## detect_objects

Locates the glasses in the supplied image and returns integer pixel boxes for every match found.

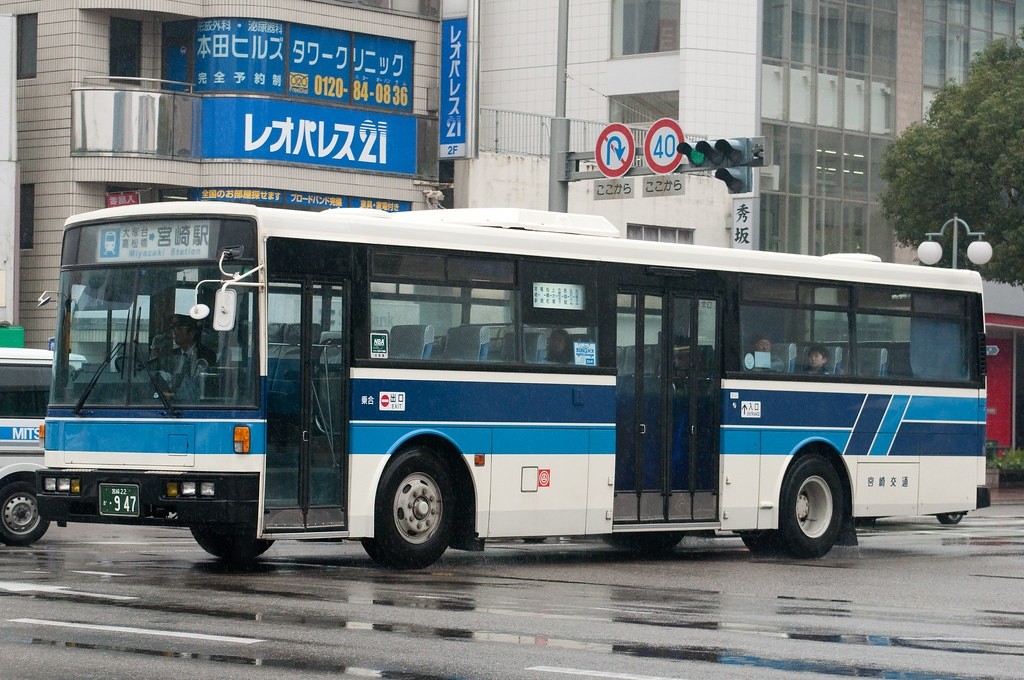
[150,345,164,350]
[171,326,191,333]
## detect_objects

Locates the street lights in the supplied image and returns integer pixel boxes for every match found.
[916,213,993,269]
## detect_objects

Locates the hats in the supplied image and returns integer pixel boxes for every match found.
[166,314,203,334]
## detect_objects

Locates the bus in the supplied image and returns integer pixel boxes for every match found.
[38,200,989,571]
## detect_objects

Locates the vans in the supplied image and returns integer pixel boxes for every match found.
[0,347,88,547]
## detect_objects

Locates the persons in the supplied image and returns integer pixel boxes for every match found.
[749,335,785,372]
[144,314,217,399]
[803,345,828,375]
[545,328,574,364]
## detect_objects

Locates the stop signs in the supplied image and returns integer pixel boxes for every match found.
[381,395,389,407]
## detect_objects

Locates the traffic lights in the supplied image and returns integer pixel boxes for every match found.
[675,137,754,195]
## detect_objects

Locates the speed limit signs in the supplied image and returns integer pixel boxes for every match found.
[643,118,684,175]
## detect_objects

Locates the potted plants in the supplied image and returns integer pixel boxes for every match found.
[986,460,1000,489]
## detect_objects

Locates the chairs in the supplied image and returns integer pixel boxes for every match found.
[801,346,842,374]
[389,324,551,363]
[268,322,341,415]
[847,348,887,377]
[616,343,715,400]
[770,343,797,373]
